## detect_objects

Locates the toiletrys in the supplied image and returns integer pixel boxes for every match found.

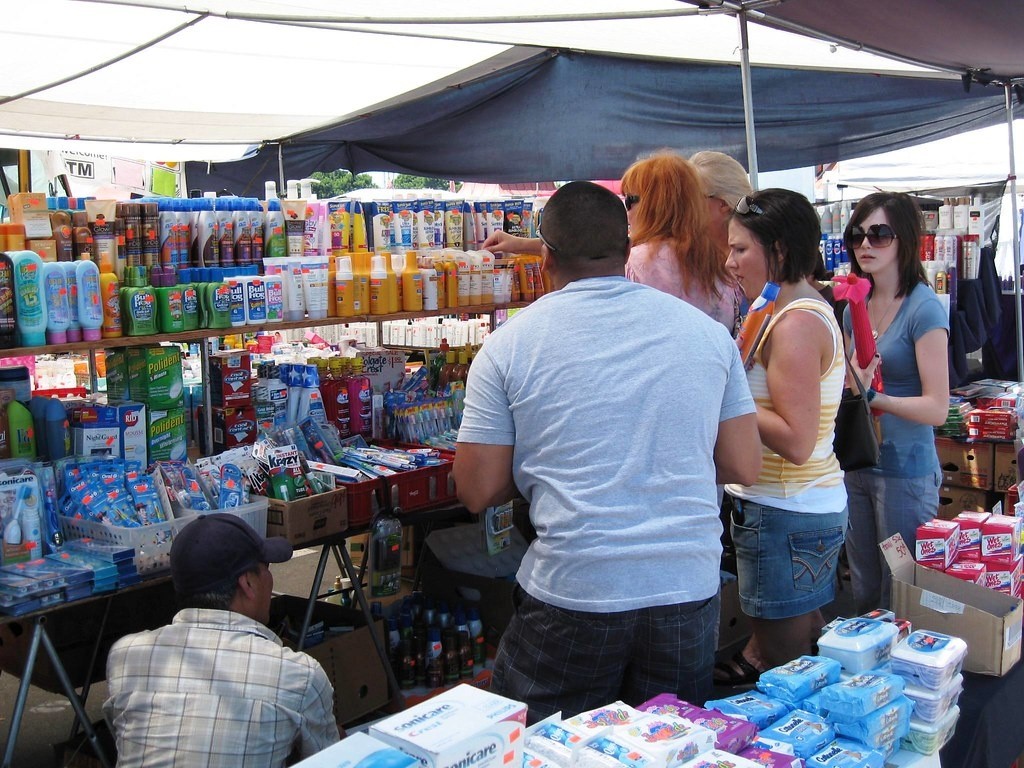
[916,197,986,310]
[816,196,853,287]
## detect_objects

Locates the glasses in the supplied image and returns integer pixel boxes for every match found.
[624,193,640,211]
[733,194,763,219]
[534,208,558,255]
[844,223,898,249]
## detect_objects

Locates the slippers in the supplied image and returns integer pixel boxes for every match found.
[712,651,767,683]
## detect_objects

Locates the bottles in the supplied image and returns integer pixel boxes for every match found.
[183,386,191,448]
[998,271,1013,290]
[433,338,472,390]
[191,385,202,446]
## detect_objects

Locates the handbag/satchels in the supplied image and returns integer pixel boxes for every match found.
[834,353,882,471]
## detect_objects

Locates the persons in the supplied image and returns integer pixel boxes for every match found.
[105,514,341,768]
[839,191,951,616]
[452,151,848,728]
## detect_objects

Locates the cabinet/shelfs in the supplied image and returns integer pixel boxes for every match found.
[0,233,966,768]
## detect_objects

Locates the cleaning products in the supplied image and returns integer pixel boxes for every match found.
[0,194,556,616]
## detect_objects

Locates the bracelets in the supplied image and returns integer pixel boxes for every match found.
[867,389,876,403]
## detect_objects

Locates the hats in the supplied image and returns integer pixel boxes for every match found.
[171,514,294,591]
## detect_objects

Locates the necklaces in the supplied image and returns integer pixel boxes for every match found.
[870,282,901,340]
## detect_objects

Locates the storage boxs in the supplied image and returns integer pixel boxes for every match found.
[262,594,526,768]
[881,534,1021,676]
[50,441,455,574]
[930,435,1015,522]
